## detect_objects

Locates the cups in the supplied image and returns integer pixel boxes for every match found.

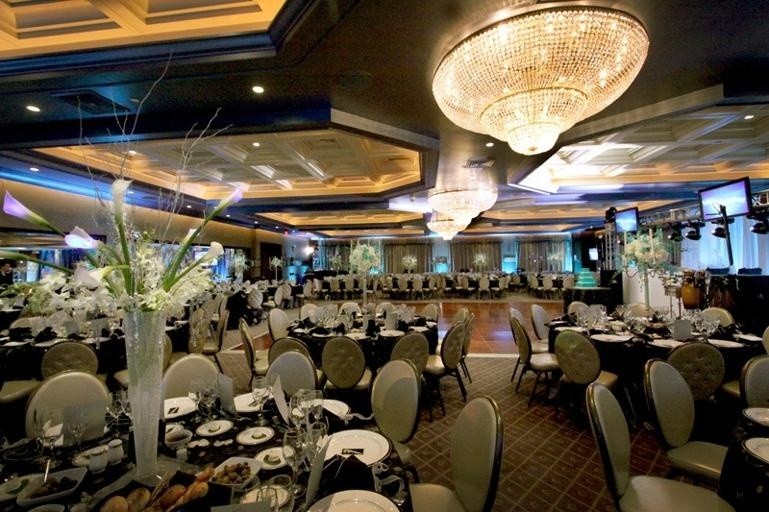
[310,391,324,422]
[268,474,295,512]
[232,475,264,505]
[303,423,329,467]
[123,390,133,421]
[106,391,127,423]
[256,488,280,512]
[62,408,89,451]
[87,448,108,475]
[108,438,124,466]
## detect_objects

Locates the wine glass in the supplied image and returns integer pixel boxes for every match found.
[36,408,66,468]
[200,379,218,422]
[288,395,310,447]
[284,428,308,500]
[188,380,205,423]
[298,388,315,427]
[550,305,763,349]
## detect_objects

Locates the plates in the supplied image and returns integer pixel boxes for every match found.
[236,426,274,446]
[163,397,197,420]
[240,484,292,510]
[16,468,87,506]
[307,490,401,512]
[319,429,393,468]
[290,303,431,340]
[1,474,41,502]
[253,379,271,425]
[206,457,262,489]
[741,437,769,464]
[291,399,351,418]
[39,423,109,447]
[71,444,110,467]
[91,478,211,512]
[1,331,125,347]
[232,385,273,412]
[256,446,296,471]
[742,407,769,427]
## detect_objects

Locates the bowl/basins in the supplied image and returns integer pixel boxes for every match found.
[165,424,185,433]
[196,420,234,436]
[166,430,194,448]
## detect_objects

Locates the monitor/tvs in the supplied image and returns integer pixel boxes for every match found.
[588,248,599,261]
[614,207,639,233]
[698,177,752,222]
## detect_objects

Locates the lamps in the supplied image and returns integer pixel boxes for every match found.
[427,186,499,241]
[431,0,651,156]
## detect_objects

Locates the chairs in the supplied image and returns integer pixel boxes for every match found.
[324,267,574,300]
[241,278,323,325]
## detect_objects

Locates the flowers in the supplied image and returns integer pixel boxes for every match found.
[1,46,253,395]
[619,226,673,273]
[348,243,381,272]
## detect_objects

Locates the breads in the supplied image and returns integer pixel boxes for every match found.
[100,465,213,512]
